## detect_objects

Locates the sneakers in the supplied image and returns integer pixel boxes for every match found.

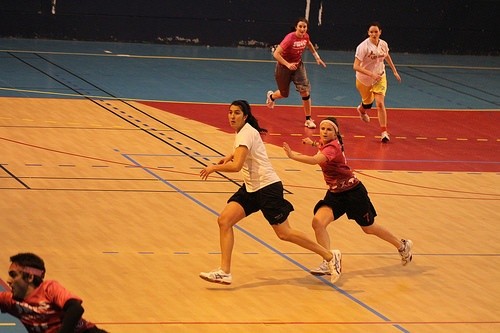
[324,250,344,283]
[357,105,370,123]
[266,90,275,110]
[381,131,390,143]
[310,260,330,276]
[398,239,413,267]
[197,267,233,285]
[305,117,316,129]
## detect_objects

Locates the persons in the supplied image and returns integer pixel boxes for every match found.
[199,99,342,285]
[266,19,326,129]
[283,117,412,276]
[304,0,323,50]
[0,253,110,333]
[353,21,400,141]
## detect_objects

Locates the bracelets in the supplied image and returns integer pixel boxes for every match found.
[312,140,316,148]
